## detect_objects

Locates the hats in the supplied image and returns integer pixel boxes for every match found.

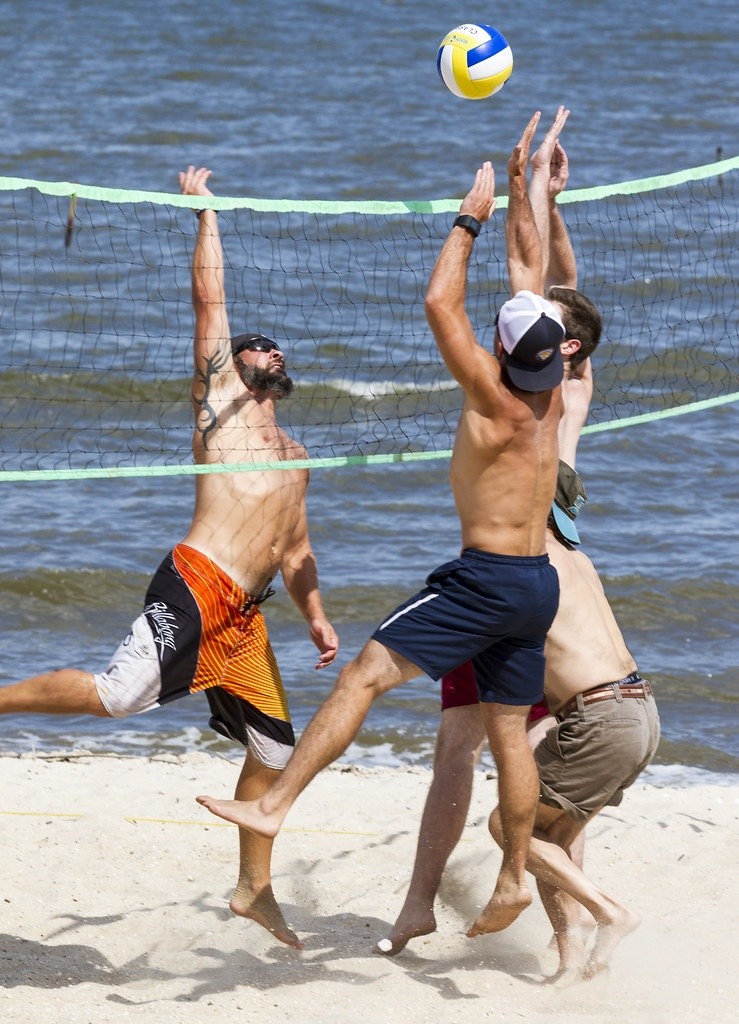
[550,458,586,545]
[498,290,566,392]
[231,334,266,351]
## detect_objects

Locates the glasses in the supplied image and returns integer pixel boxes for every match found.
[234,337,281,359]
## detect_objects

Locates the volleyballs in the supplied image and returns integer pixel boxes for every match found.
[437,24,513,100]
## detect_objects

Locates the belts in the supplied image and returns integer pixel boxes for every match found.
[558,681,653,723]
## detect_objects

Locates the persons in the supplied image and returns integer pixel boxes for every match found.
[197,111,566,936]
[2,164,340,951]
[372,102,604,956]
[490,458,660,988]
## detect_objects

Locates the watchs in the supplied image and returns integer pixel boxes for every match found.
[453,214,483,239]
